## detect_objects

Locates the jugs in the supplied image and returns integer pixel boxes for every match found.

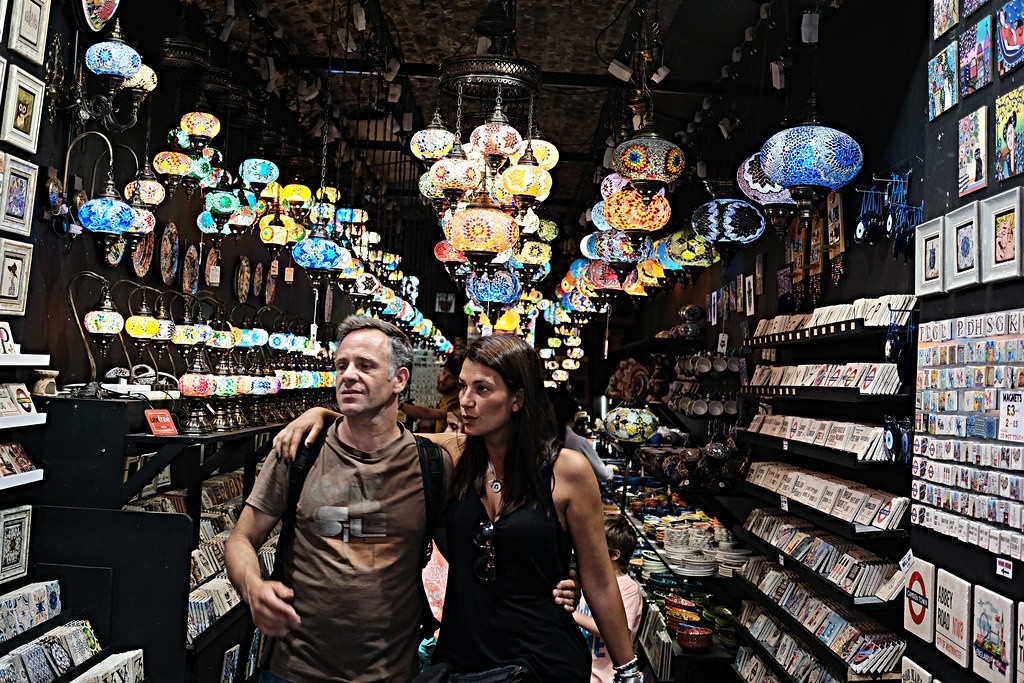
[641,503,687,516]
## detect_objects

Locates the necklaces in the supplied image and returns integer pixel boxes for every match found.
[488,460,503,492]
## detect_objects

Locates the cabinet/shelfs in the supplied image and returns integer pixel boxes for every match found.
[600,305,920,683]
[0,392,292,683]
[0,354,50,489]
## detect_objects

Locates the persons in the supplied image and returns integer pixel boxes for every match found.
[225,313,581,683]
[271,334,644,683]
[553,399,619,482]
[571,513,643,683]
[398,352,468,663]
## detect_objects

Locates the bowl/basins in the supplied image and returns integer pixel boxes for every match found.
[629,544,743,652]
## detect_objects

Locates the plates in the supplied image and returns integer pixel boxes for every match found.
[644,510,752,576]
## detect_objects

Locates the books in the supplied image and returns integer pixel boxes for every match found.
[733,294,1024,683]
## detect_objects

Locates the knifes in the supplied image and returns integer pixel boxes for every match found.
[713,296,715,321]
[747,280,752,311]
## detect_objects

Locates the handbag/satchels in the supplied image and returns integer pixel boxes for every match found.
[440,665,528,683]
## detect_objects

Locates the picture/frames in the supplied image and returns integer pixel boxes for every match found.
[0,153,39,237]
[1,64,46,155]
[0,237,34,316]
[915,215,944,298]
[944,200,979,293]
[8,0,50,66]
[0,504,33,583]
[981,186,1023,284]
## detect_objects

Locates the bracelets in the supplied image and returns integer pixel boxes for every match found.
[398,403,403,410]
[612,654,644,683]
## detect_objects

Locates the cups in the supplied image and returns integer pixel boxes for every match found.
[668,351,740,416]
[635,445,681,470]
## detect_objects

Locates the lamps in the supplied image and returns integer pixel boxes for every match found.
[46,0,866,398]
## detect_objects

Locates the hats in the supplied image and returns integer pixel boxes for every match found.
[440,359,462,376]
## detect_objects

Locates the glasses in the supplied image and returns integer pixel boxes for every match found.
[472,522,496,582]
